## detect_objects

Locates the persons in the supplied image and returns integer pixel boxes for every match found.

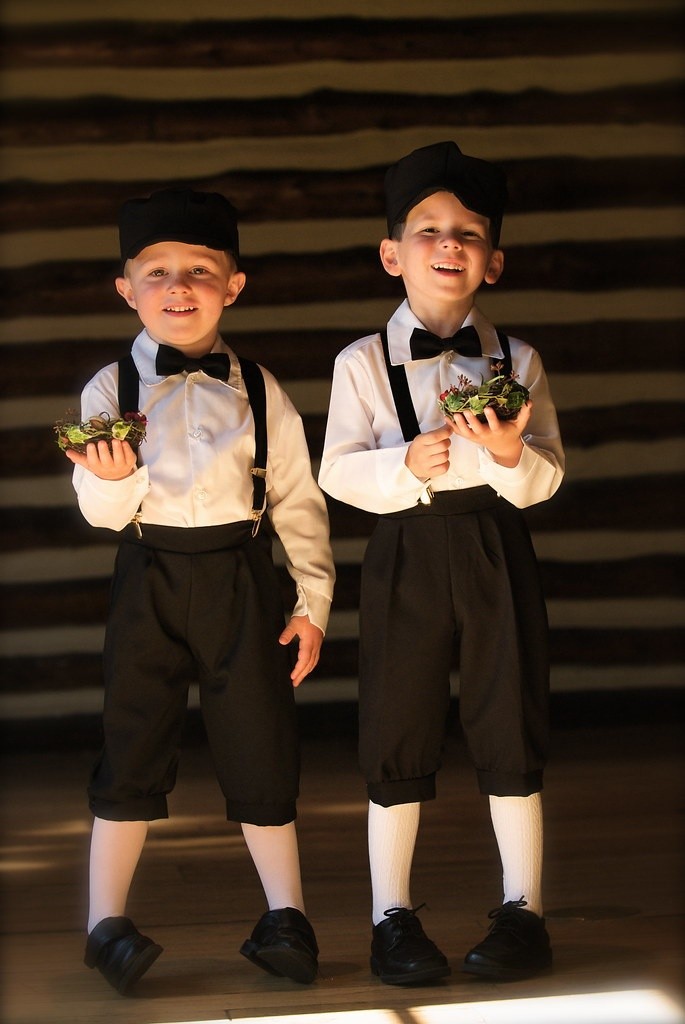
[65,192,338,993]
[316,140,565,987]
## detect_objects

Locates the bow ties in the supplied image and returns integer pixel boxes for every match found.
[155,344,231,384]
[410,324,483,362]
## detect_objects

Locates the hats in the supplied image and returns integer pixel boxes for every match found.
[381,140,512,247]
[115,185,239,274]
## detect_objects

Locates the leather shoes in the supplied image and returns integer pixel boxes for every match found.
[463,895,553,971]
[239,907,319,986]
[369,901,451,984]
[82,916,162,994]
